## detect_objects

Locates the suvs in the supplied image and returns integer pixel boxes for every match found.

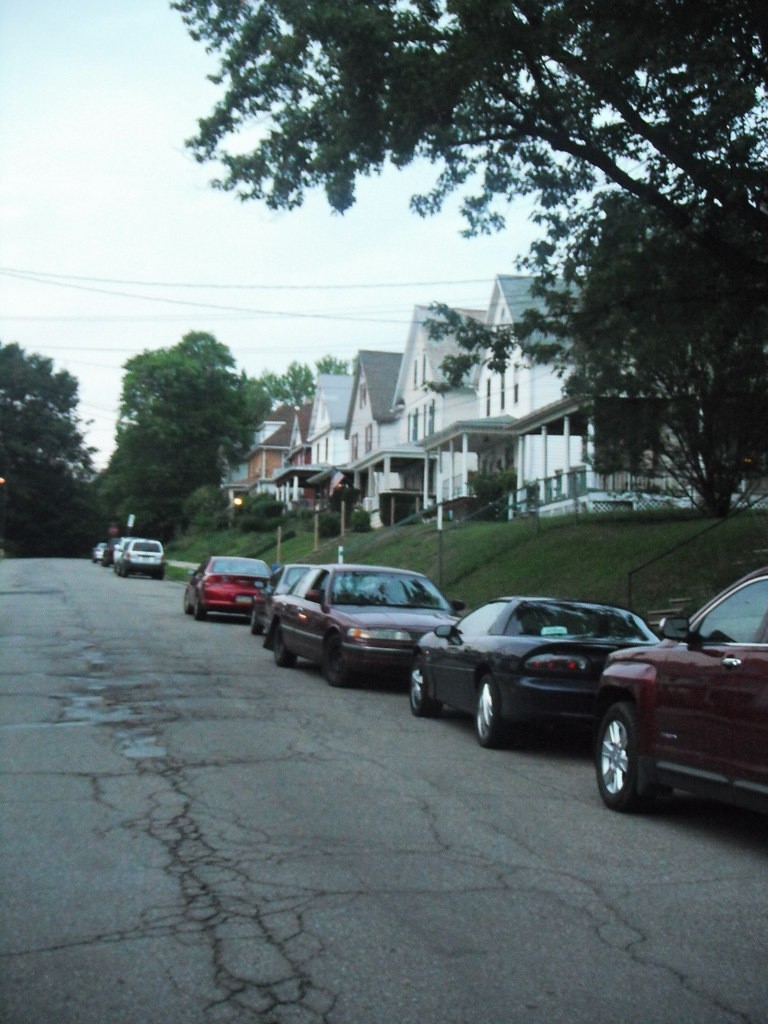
[116,539,166,579]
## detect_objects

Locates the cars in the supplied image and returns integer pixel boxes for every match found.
[182,556,273,620]
[249,566,314,632]
[266,564,465,689]
[409,593,659,748]
[590,564,768,817]
[92,537,135,567]
